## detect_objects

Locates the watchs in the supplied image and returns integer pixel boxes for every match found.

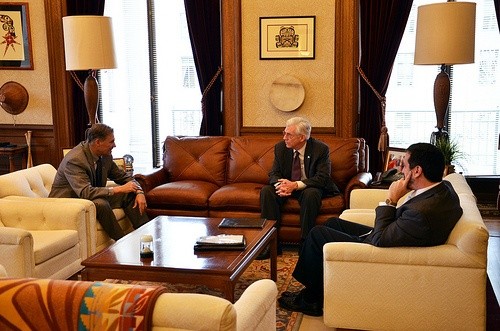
[385,198,397,206]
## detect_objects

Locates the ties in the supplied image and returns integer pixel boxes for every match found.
[95,160,102,186]
[292,151,301,181]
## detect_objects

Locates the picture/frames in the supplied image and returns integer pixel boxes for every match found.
[259,15,315,60]
[0,1,34,70]
[383,145,408,172]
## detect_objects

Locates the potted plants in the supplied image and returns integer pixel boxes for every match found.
[432,134,466,177]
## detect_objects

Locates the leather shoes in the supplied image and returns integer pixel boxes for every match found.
[278,291,324,317]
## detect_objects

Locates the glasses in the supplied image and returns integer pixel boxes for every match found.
[283,131,301,138]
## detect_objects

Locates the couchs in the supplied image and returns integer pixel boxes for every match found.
[1,134,489,330]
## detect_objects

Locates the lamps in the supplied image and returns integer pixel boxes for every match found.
[413,0,476,134]
[62,15,116,131]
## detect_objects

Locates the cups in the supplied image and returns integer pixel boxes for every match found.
[140,235,153,262]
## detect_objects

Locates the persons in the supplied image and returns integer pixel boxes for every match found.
[254,116,339,261]
[48,122,149,241]
[278,142,463,316]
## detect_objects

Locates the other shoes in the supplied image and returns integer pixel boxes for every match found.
[256,246,283,260]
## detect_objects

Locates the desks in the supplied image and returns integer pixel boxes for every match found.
[80,215,278,304]
[0,144,28,174]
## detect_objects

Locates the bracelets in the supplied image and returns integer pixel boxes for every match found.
[137,191,144,194]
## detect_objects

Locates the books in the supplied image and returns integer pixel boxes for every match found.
[219,218,266,228]
[194,234,246,250]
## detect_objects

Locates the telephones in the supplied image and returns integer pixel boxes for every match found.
[381,168,404,185]
